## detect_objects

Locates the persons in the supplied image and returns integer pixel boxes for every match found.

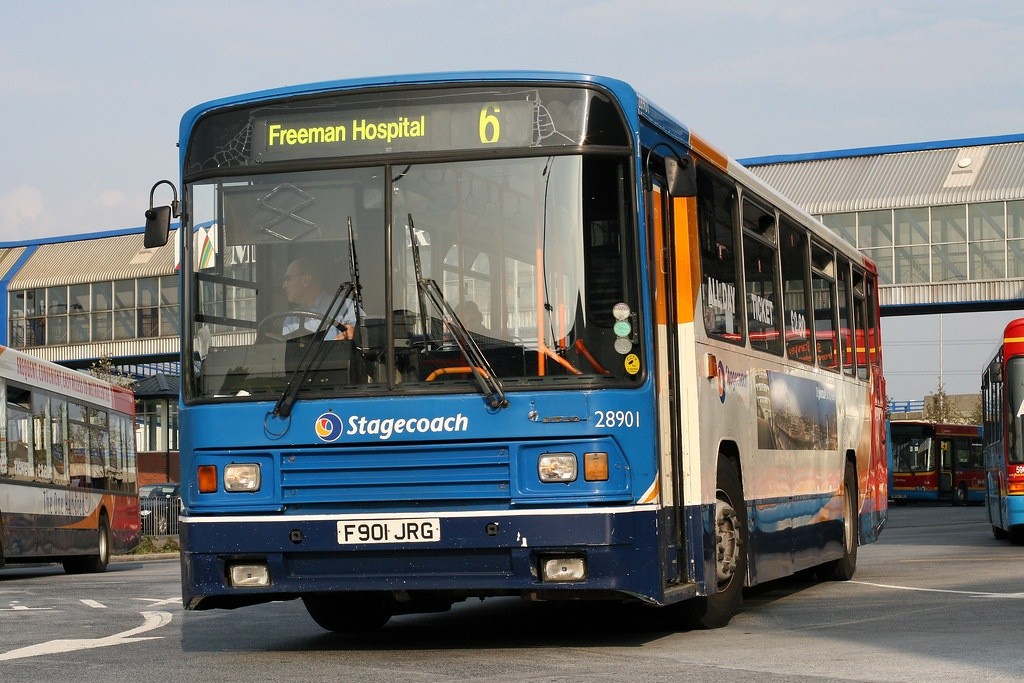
[283,256,367,340]
[454,301,491,336]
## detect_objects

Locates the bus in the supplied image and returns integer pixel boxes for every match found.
[723,329,877,383]
[981,318,1024,546]
[0,344,142,575]
[143,70,890,630]
[890,421,986,507]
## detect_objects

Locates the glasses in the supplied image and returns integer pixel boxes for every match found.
[284,272,304,280]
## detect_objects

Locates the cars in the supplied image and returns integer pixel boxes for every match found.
[138,483,180,535]
